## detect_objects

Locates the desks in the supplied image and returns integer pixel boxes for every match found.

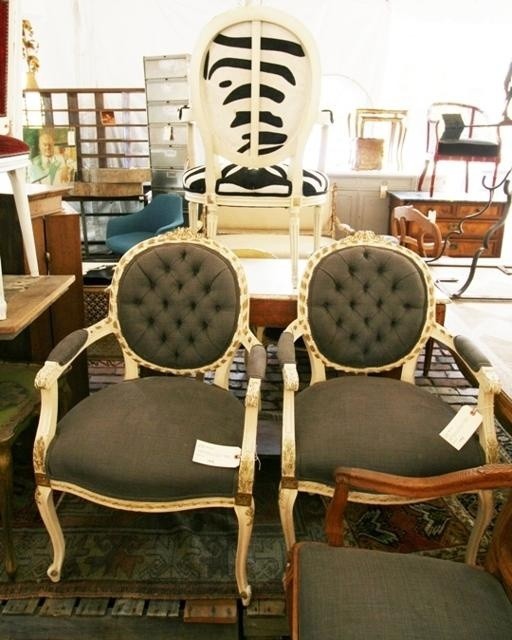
[344,108,409,174]
[0,359,73,578]
[239,256,447,383]
[0,275,76,339]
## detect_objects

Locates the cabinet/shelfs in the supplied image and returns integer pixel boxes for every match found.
[142,54,197,200]
[22,87,150,262]
[389,189,508,259]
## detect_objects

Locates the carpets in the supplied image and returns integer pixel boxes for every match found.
[0,404,511,599]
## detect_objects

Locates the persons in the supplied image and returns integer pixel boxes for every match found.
[31,133,67,186]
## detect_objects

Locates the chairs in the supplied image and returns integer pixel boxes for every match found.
[418,100,502,197]
[33,226,269,605]
[181,9,333,287]
[275,230,502,564]
[0,9,42,321]
[282,463,512,640]
